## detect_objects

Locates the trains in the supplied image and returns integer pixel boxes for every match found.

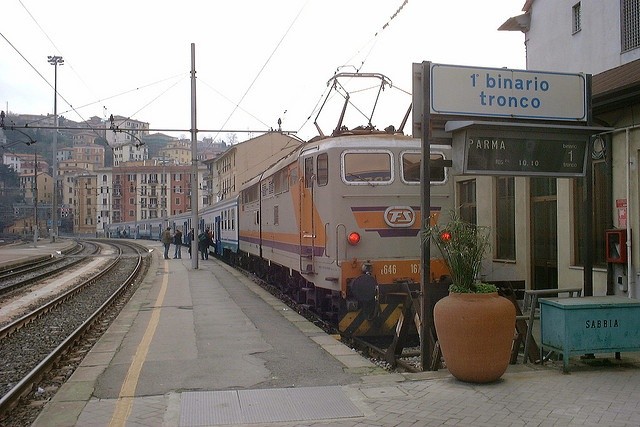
[104,129,456,338]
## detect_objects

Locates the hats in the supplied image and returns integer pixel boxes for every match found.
[167,227,171,229]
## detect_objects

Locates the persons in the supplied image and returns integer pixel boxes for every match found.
[161,227,173,259]
[188,230,192,259]
[198,228,212,260]
[206,227,213,249]
[173,229,182,259]
[123,230,126,238]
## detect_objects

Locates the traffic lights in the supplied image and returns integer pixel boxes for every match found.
[65,207,69,217]
[61,207,65,218]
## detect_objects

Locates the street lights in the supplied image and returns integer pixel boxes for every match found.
[47,53,64,243]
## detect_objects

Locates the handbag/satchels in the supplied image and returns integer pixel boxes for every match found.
[199,232,206,242]
